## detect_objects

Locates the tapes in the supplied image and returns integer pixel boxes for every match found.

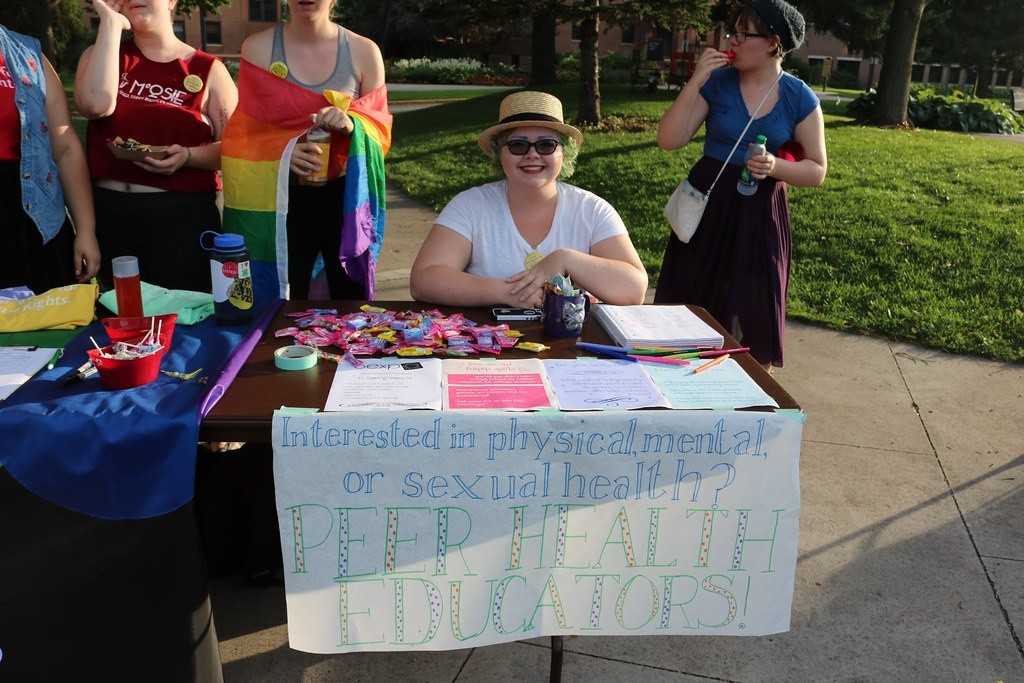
[274,345,318,370]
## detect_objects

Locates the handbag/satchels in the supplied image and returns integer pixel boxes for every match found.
[664,180,709,243]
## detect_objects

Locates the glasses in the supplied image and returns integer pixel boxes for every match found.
[502,138,561,155]
[724,30,764,43]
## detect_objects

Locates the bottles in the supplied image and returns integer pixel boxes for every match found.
[301,112,330,186]
[201,231,255,327]
[737,135,767,196]
[112,256,144,318]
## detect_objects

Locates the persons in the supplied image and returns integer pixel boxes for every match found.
[73,0,239,293]
[410,91,648,309]
[241,0,385,299]
[654,0,827,373]
[0,25,101,295]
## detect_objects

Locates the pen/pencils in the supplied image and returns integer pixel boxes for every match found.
[581,345,638,362]
[626,354,691,365]
[700,348,750,357]
[627,345,718,357]
[575,341,628,352]
[65,367,98,385]
[68,361,92,378]
[47,348,61,371]
[662,351,714,358]
[693,353,731,374]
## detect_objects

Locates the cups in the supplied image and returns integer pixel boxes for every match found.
[545,290,590,338]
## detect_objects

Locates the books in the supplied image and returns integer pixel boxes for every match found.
[591,304,724,349]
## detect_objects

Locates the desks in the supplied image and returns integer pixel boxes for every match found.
[0,299,803,683]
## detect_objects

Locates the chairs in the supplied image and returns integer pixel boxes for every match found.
[666,51,696,92]
[629,49,661,92]
[647,37,671,83]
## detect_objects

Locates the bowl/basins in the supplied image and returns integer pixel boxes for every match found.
[86,333,166,389]
[100,313,178,353]
[107,140,168,160]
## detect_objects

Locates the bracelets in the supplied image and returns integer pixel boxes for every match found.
[185,147,191,166]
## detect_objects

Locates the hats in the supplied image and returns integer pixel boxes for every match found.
[751,1,806,51]
[478,91,583,159]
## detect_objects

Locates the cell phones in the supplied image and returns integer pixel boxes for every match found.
[492,307,543,321]
